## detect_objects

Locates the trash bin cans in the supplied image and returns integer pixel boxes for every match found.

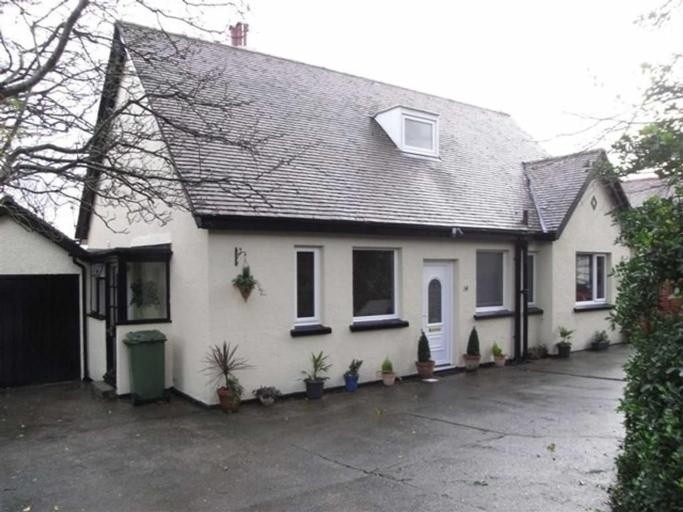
[122,329,171,406]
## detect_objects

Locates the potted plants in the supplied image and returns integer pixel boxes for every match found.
[252,384,286,409]
[551,324,578,359]
[229,244,266,305]
[340,357,364,394]
[459,325,484,373]
[376,352,404,388]
[588,330,613,352]
[413,327,438,381]
[194,338,261,415]
[487,340,510,368]
[300,349,330,401]
